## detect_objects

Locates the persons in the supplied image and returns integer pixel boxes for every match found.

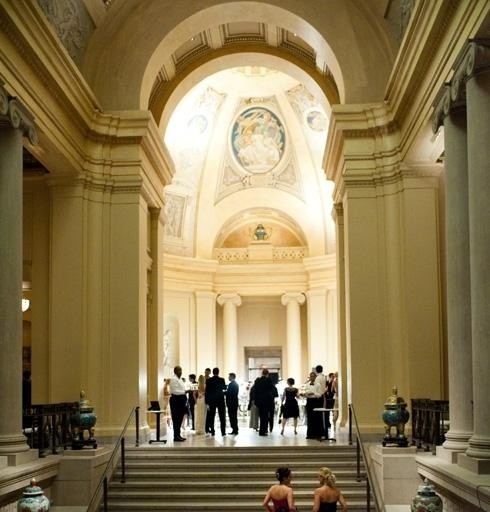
[312,467,347,512]
[163,366,339,441]
[262,467,298,512]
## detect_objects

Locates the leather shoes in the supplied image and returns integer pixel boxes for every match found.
[207,429,238,436]
[180,436,186,440]
[175,436,183,441]
